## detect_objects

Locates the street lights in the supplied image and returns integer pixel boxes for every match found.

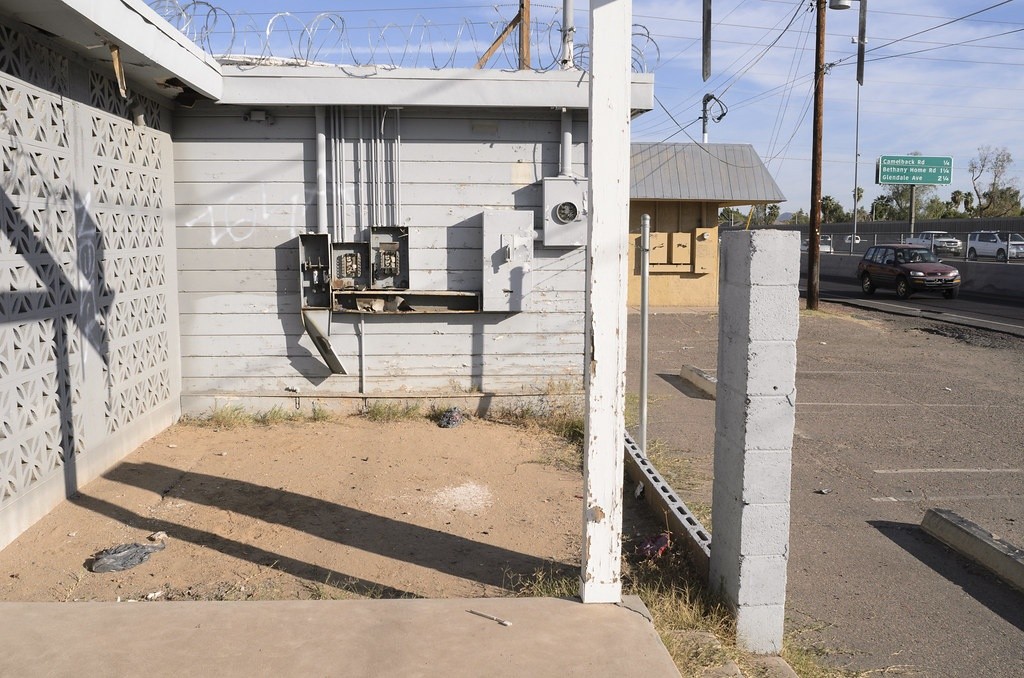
[806,0,852,311]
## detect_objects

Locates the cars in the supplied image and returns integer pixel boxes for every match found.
[800,234,834,255]
[845,235,861,244]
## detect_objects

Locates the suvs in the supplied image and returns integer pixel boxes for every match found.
[856,244,961,301]
[968,230,1024,262]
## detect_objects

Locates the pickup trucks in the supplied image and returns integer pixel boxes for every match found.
[906,230,963,256]
